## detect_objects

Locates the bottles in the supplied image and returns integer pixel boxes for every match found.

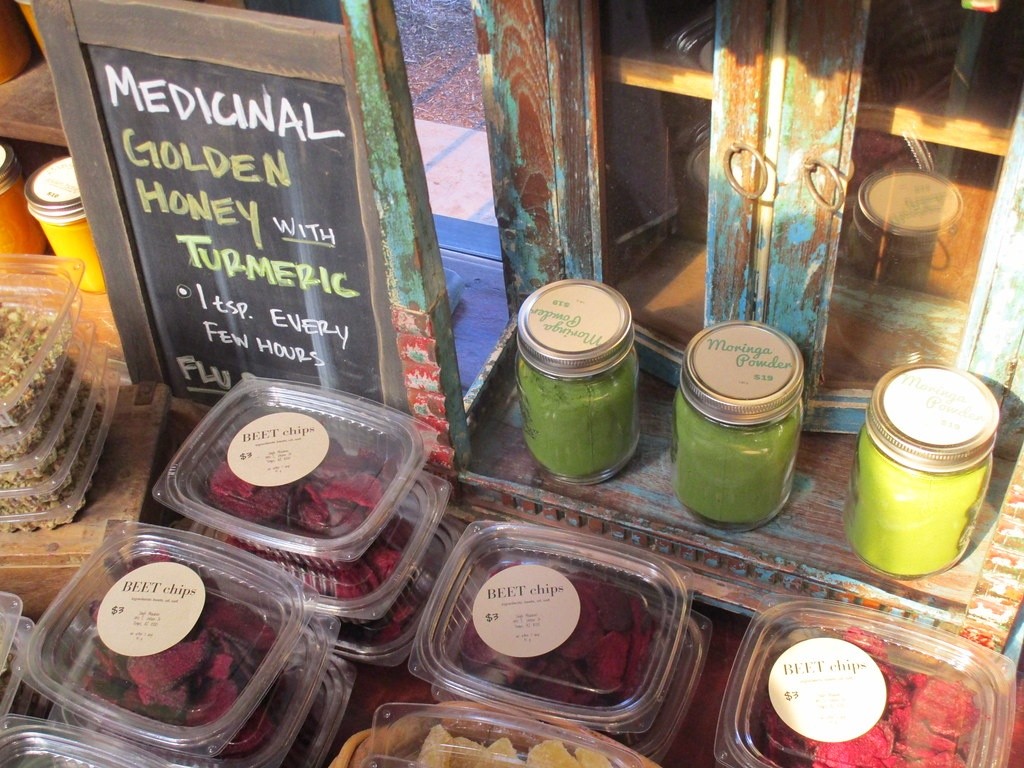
[671,320,807,532]
[1,0,110,296]
[845,360,1001,580]
[515,278,643,485]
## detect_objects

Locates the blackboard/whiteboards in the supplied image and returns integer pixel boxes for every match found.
[34,0,406,410]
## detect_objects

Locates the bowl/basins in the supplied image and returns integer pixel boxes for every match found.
[0,246,1019,768]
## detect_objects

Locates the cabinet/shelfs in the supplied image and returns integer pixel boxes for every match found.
[475,0,1024,434]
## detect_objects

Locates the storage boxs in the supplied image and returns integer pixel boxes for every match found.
[0,251,1017,768]
